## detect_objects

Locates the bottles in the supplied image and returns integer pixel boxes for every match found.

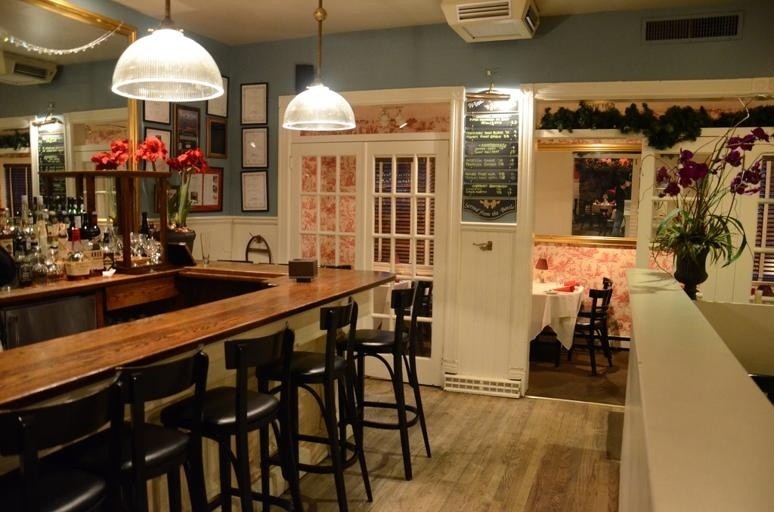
[14,194,96,249]
[68,227,114,277]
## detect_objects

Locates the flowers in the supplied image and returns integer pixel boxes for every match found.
[90,138,134,170]
[648,125,769,278]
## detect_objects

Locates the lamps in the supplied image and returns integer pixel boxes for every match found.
[33,103,59,128]
[107,0,224,104]
[281,1,357,132]
[465,66,511,102]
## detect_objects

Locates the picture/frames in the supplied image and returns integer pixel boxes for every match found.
[138,72,271,215]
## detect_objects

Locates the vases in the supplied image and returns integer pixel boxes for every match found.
[672,237,710,299]
[155,230,195,266]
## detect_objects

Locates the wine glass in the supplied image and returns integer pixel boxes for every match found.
[116,231,163,265]
[32,246,63,284]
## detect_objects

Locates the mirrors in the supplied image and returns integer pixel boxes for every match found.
[0,0,142,268]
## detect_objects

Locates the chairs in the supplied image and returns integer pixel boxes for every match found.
[556,276,612,377]
